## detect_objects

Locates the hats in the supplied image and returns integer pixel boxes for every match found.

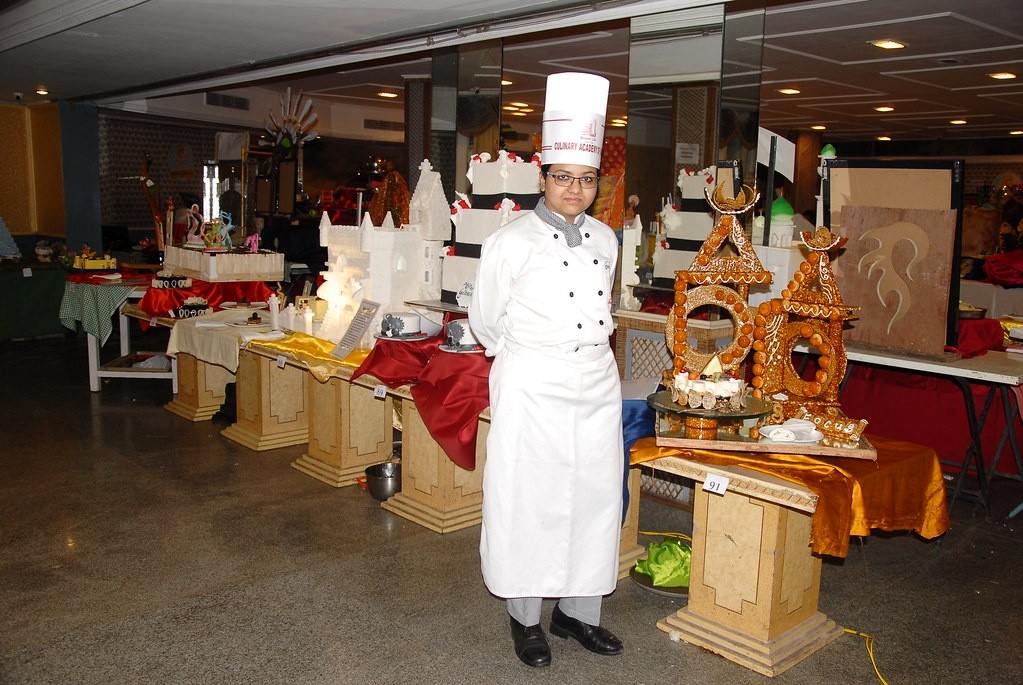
[541,72,610,169]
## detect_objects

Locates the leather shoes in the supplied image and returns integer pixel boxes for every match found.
[549,602,623,656]
[509,615,552,668]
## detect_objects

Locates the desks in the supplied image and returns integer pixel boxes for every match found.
[785,334,1023,522]
[65,270,263,394]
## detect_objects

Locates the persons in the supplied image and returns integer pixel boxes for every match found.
[467,142,624,668]
[289,199,328,287]
[1001,190,1023,235]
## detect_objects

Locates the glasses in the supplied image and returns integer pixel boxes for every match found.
[547,172,599,189]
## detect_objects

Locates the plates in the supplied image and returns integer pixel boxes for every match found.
[958,307,988,320]
[224,317,273,328]
[1003,313,1023,324]
[759,424,825,444]
[218,300,268,311]
